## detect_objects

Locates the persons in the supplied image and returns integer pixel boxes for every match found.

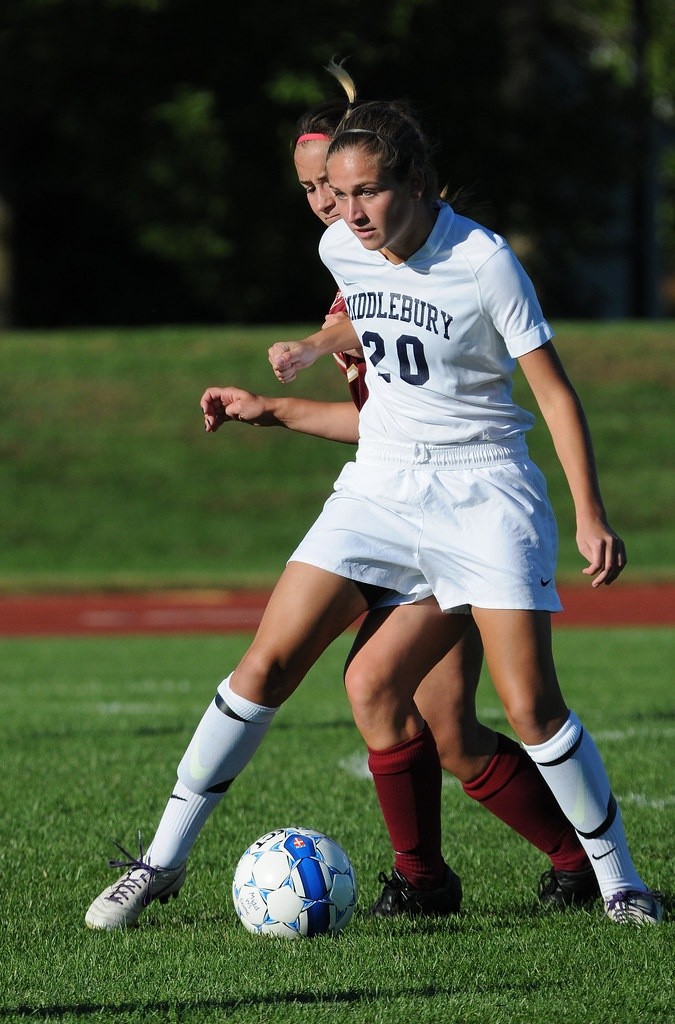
[75,91,673,933]
[200,106,607,927]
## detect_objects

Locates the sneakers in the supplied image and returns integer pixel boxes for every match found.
[531,865,600,912]
[603,885,668,929]
[84,829,187,930]
[366,869,463,917]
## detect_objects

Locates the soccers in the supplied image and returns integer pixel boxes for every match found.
[232,826,357,939]
